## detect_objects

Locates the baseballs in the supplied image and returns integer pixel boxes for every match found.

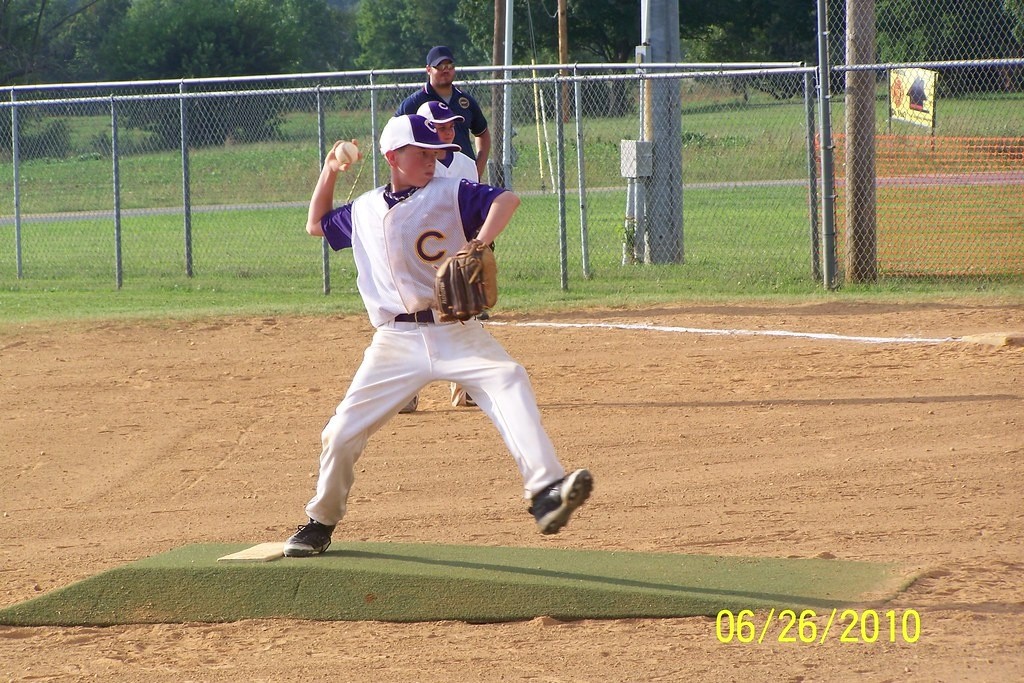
[334,142,359,164]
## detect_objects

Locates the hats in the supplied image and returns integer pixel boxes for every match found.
[379,114,462,156]
[416,101,465,124]
[426,46,454,67]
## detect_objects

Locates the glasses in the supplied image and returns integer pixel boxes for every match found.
[433,63,455,71]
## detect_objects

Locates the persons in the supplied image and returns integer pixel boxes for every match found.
[398,101,479,411]
[283,115,594,560]
[396,46,489,184]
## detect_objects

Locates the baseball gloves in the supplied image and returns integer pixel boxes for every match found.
[435,237,498,318]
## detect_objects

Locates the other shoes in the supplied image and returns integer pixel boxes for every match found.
[476,312,489,320]
[398,396,418,413]
[466,392,477,406]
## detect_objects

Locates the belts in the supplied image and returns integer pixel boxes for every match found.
[395,310,434,324]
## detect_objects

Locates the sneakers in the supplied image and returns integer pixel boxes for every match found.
[283,517,336,557]
[528,469,593,535]
